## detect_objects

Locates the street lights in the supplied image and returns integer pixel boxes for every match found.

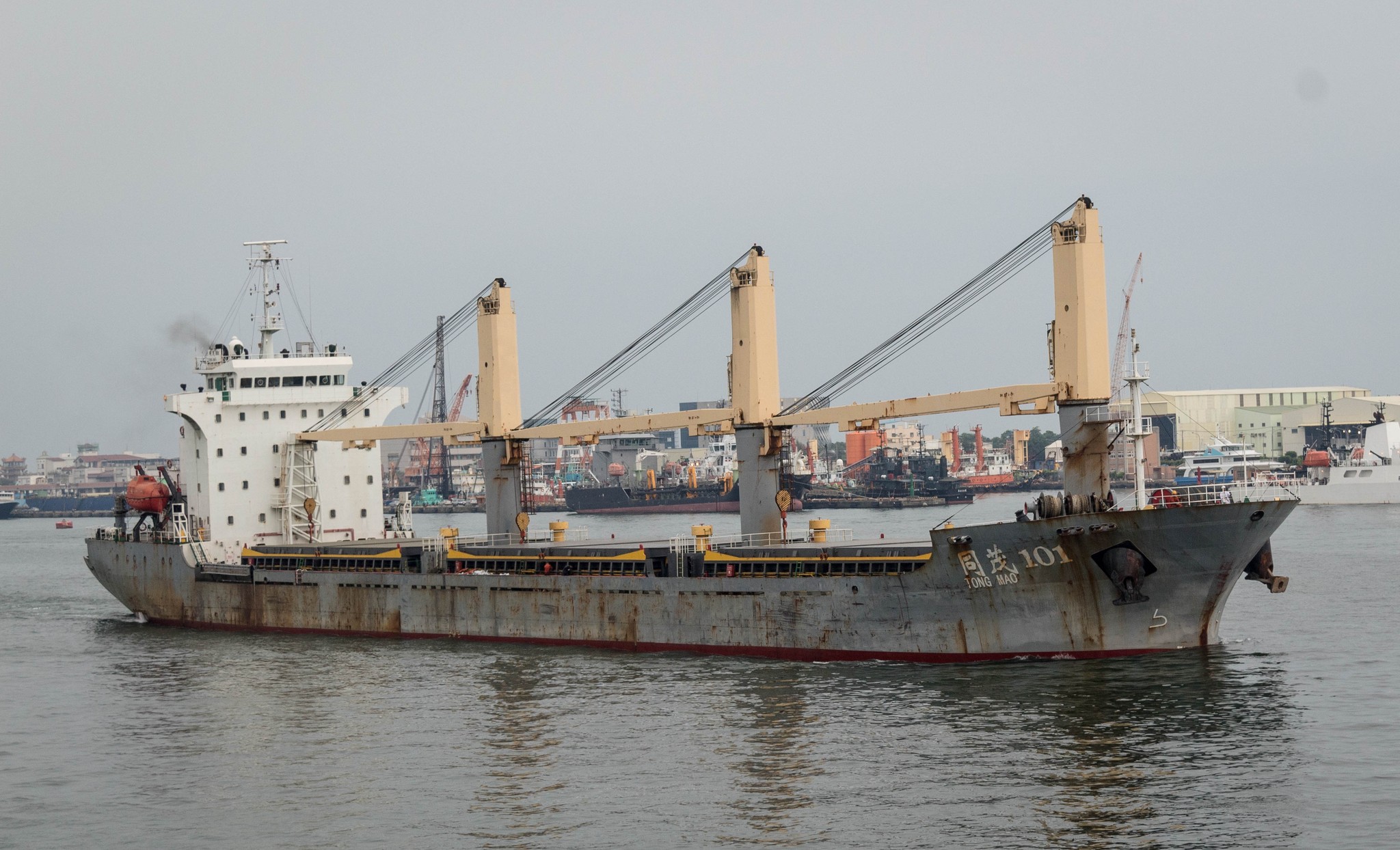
[1191,432,1201,452]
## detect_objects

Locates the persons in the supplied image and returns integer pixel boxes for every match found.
[1170,491,1182,507]
[544,562,552,575]
[1220,485,1232,504]
[561,564,573,576]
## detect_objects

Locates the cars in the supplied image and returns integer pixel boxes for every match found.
[388,500,399,506]
[451,499,465,506]
[437,500,451,505]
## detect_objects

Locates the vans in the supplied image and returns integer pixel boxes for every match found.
[465,497,478,504]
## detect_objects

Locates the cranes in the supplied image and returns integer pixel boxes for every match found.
[407,372,474,482]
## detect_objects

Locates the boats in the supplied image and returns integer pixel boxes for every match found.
[1229,398,1400,504]
[964,477,1033,494]
[78,237,1309,661]
[1174,426,1298,485]
[26,481,131,512]
[852,422,975,504]
[0,491,18,518]
[563,462,814,514]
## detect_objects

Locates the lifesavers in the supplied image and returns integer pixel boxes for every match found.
[1299,466,1303,470]
[1373,461,1376,466]
[180,427,184,436]
[1296,466,1299,470]
[167,459,172,468]
[140,523,147,531]
